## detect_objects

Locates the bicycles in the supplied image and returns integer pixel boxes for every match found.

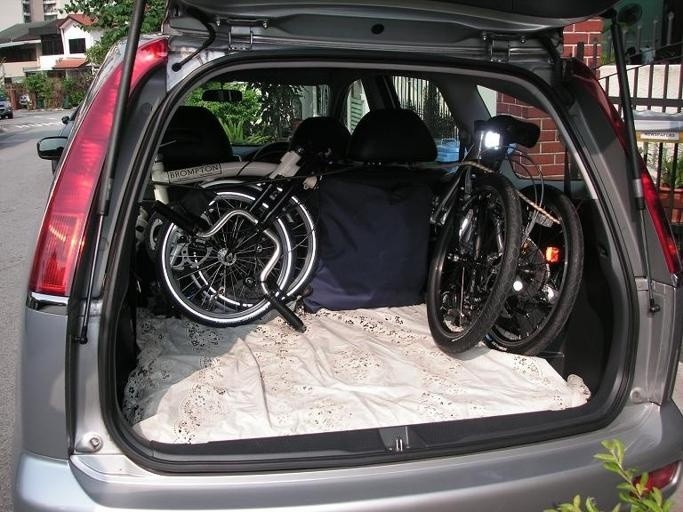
[424,116,584,356]
[144,137,337,333]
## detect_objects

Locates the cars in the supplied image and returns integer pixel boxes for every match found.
[14,0,683,512]
[0,95,13,119]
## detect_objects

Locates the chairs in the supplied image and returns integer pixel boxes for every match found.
[160,105,450,308]
